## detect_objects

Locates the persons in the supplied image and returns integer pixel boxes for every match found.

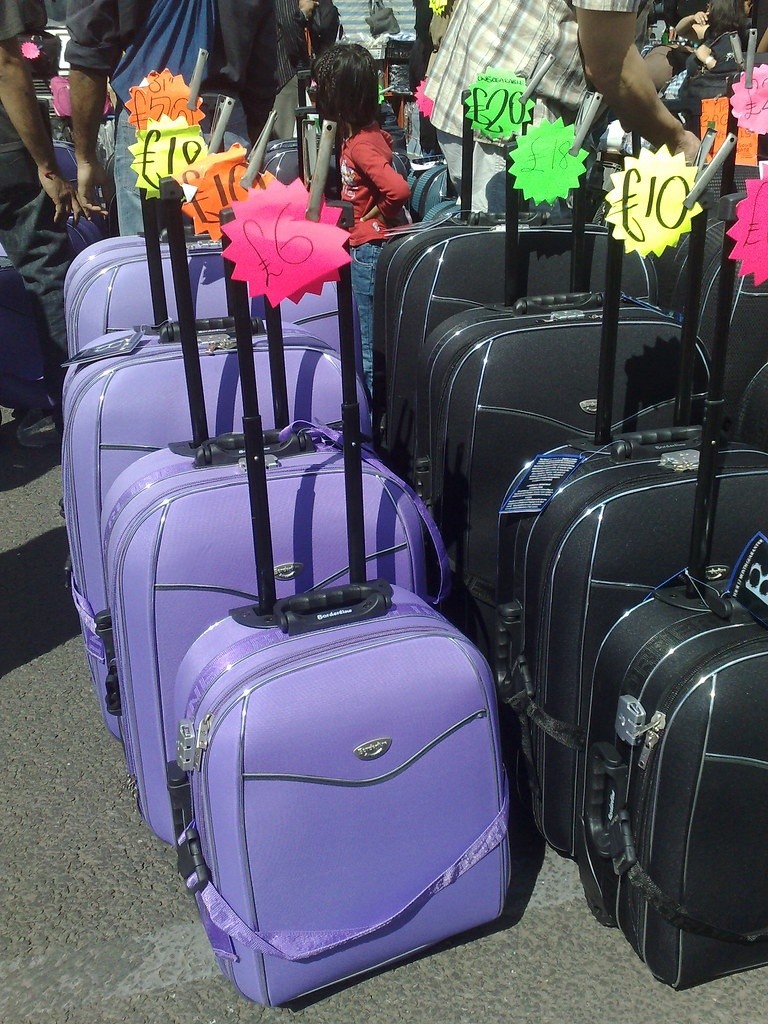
[0,0,768,438]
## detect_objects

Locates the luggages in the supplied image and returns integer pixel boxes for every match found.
[46,66,767,1009]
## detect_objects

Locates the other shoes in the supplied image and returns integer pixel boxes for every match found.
[17,415,59,450]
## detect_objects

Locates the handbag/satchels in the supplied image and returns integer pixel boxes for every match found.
[50,75,113,122]
[365,1,400,39]
